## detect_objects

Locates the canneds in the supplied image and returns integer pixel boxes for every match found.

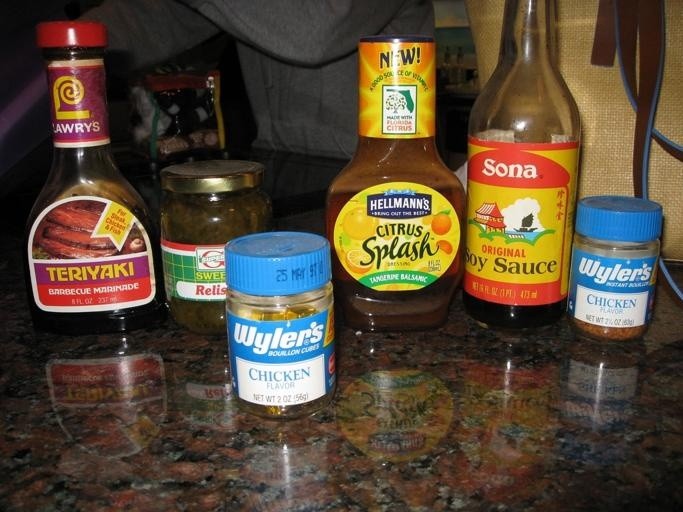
[158,159,279,336]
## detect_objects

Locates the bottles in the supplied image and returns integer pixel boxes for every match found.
[463,2,584,324]
[21,20,165,336]
[17,328,654,510]
[320,35,463,333]
